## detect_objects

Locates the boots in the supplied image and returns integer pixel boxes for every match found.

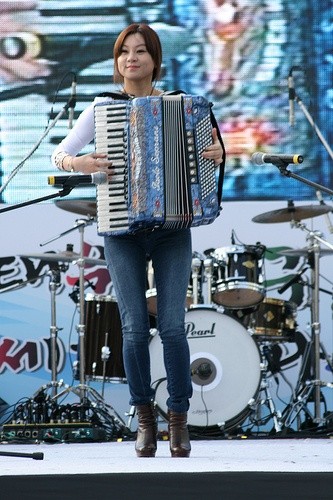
[168,409,191,457]
[135,401,157,457]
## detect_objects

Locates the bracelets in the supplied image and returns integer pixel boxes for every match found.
[68,157,78,173]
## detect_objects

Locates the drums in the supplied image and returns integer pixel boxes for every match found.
[208,243,267,309]
[74,292,130,384]
[144,306,265,437]
[222,297,298,342]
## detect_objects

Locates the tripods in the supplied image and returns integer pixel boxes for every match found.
[15,213,129,441]
[241,215,333,434]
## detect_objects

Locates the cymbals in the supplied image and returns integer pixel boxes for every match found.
[250,204,333,224]
[53,196,98,217]
[15,253,108,266]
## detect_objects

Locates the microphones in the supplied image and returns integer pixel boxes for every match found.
[251,152,303,165]
[316,190,333,233]
[288,76,295,127]
[68,75,77,129]
[48,172,109,187]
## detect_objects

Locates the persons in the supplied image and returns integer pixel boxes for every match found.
[51,24,223,457]
